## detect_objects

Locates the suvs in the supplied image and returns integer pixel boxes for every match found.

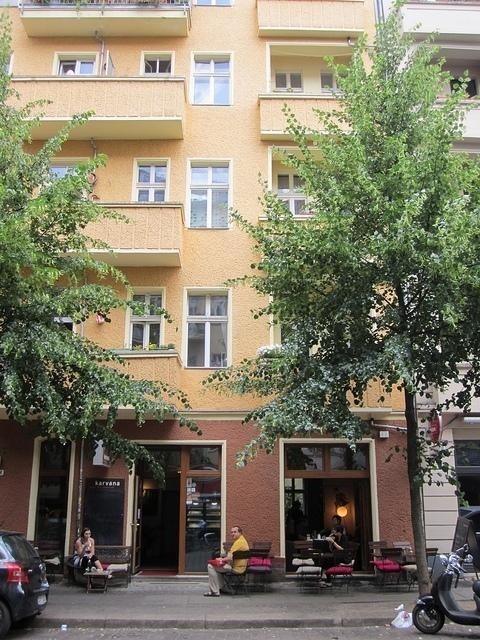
[0,530,50,640]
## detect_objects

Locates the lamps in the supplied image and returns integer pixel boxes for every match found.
[335,487,350,517]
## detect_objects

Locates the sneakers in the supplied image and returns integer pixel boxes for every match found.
[105,571,112,579]
[320,581,332,587]
[204,589,222,596]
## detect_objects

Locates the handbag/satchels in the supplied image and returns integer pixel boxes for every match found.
[72,554,88,567]
[208,560,226,567]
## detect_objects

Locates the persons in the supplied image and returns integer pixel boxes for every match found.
[204,526,249,597]
[329,515,344,537]
[321,525,352,588]
[73,527,112,579]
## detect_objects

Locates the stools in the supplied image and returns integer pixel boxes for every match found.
[83,571,112,595]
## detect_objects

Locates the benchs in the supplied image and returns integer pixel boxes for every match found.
[69,546,133,588]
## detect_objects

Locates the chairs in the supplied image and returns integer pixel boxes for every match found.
[369,541,438,592]
[291,535,359,595]
[213,542,274,598]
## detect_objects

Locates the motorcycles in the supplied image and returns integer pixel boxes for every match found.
[412,544,480,633]
